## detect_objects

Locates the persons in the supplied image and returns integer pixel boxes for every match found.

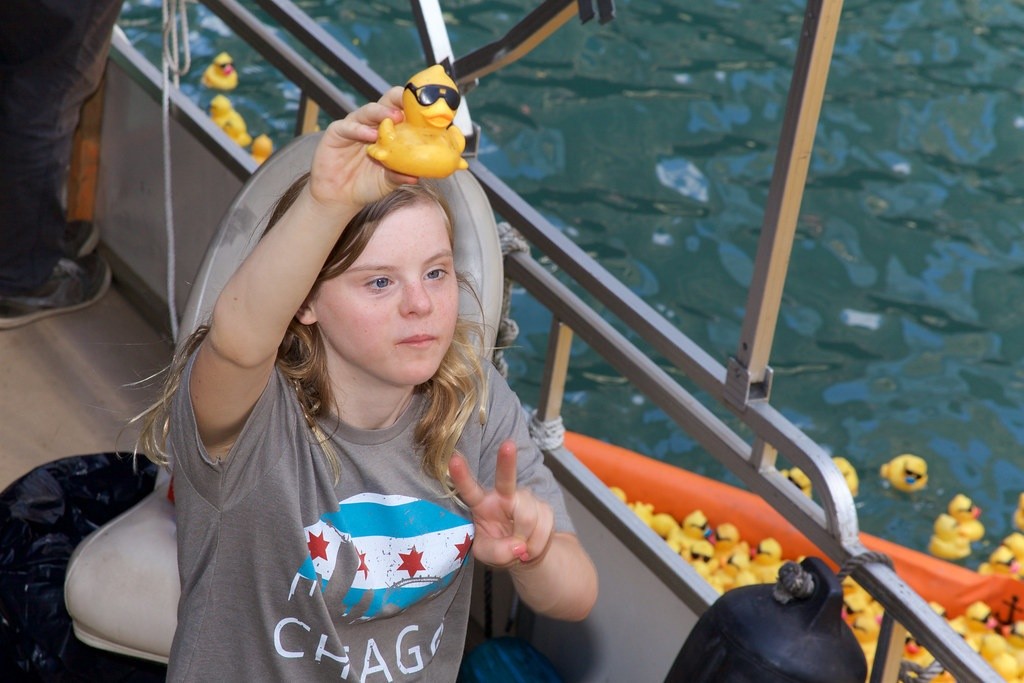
[113,87,597,683]
[1,1,112,334]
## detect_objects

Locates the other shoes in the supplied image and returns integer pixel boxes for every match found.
[0,217,114,330]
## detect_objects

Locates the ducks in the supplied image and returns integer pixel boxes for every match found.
[605,453,1024,683]
[198,51,273,167]
[368,65,469,180]
